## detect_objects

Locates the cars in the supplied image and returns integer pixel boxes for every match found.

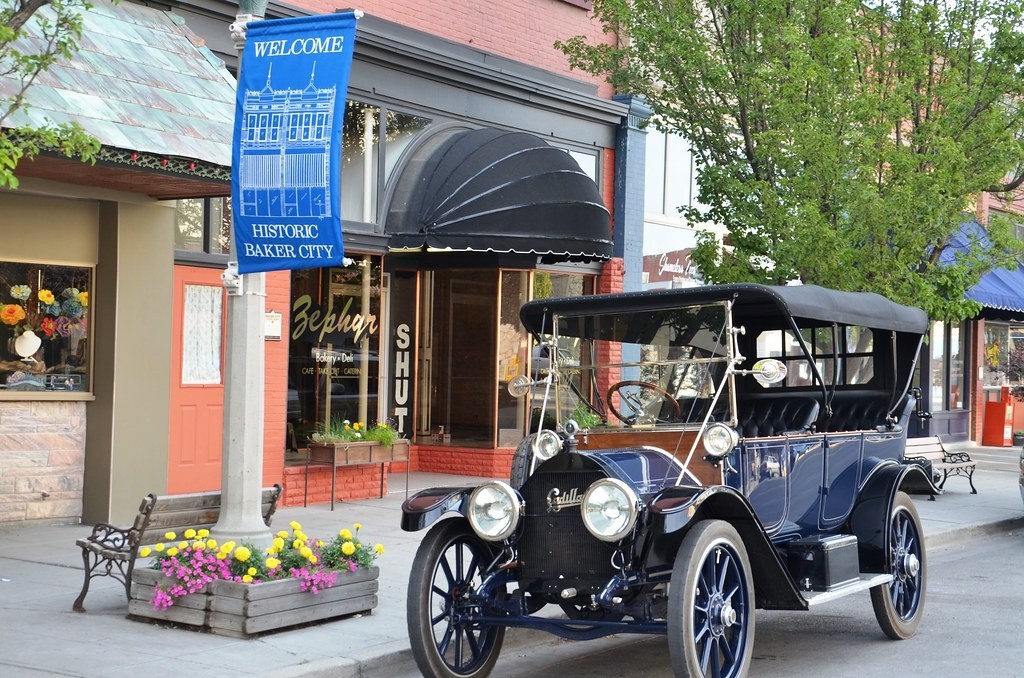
[398,282,948,676]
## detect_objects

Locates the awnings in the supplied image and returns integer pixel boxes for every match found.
[384,128,614,265]
[838,208,1024,322]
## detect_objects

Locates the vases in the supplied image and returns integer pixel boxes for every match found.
[125,565,379,639]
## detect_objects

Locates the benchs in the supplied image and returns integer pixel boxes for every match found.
[73,484,283,613]
[702,390,898,439]
[904,434,976,501]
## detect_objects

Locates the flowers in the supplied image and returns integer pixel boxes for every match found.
[140,520,384,613]
[307,410,399,449]
[0,284,88,338]
